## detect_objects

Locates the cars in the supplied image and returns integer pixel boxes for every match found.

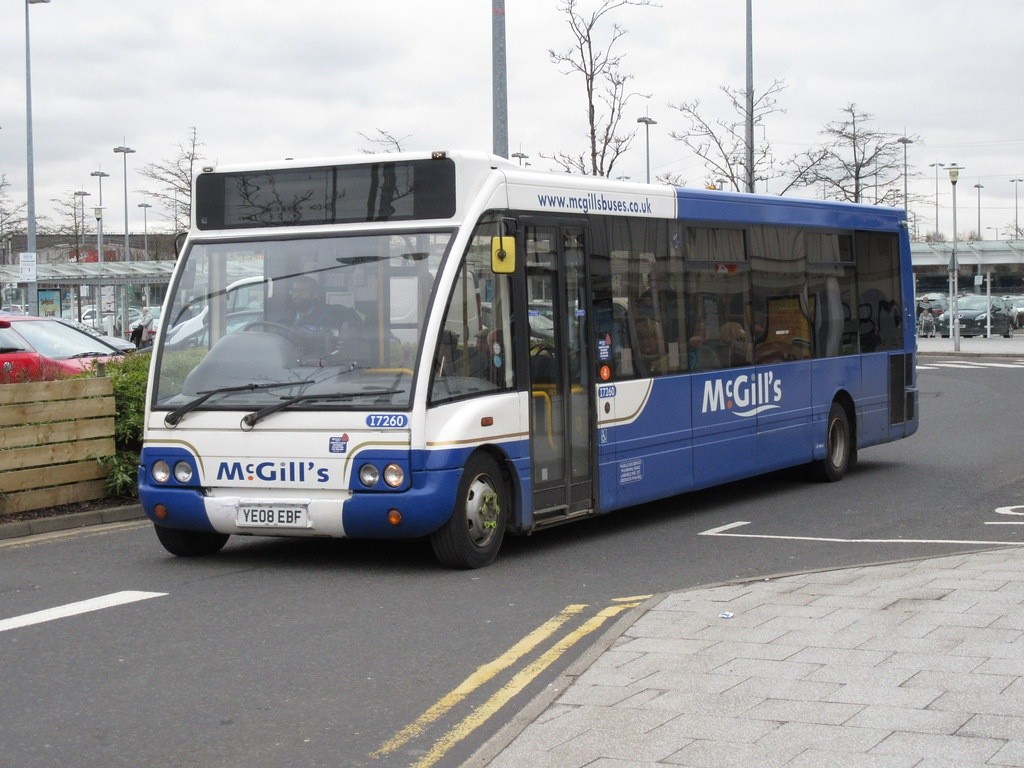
[915,289,1024,338]
[0,299,208,385]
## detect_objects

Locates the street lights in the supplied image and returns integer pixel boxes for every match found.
[942,163,964,352]
[929,161,945,239]
[974,184,984,241]
[73,191,91,263]
[637,117,658,185]
[897,136,913,212]
[112,145,135,261]
[1009,177,1024,240]
[89,205,107,334]
[136,204,153,307]
[90,171,109,262]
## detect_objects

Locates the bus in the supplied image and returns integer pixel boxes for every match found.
[137,152,921,570]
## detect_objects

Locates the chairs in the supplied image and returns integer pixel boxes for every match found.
[630,310,825,371]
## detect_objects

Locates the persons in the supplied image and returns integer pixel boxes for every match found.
[918,310,935,339]
[917,295,933,323]
[125,307,153,350]
[275,273,345,364]
[438,268,899,390]
